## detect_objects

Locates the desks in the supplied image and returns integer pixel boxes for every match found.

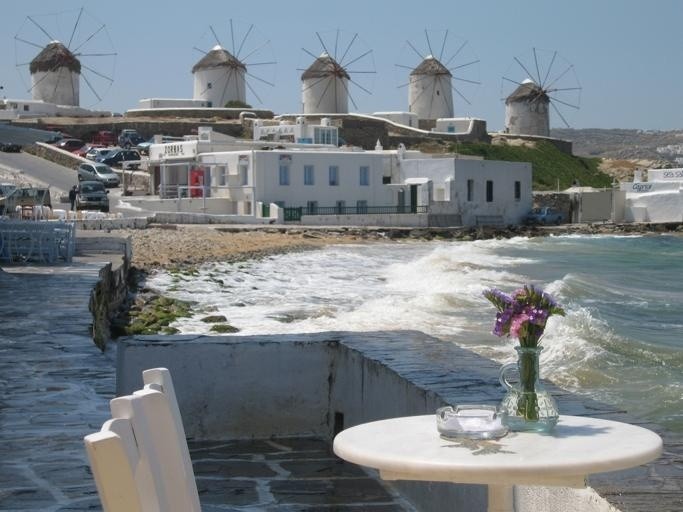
[331,412,664,511]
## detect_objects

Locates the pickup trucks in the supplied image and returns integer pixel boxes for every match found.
[521,206,568,226]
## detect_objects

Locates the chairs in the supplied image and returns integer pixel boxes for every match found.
[79,366,204,511]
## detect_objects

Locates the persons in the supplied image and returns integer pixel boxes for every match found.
[69,185,78,210]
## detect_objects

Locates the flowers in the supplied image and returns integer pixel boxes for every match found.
[482,284,567,419]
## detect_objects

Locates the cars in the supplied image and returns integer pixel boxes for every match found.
[0,128,173,212]
